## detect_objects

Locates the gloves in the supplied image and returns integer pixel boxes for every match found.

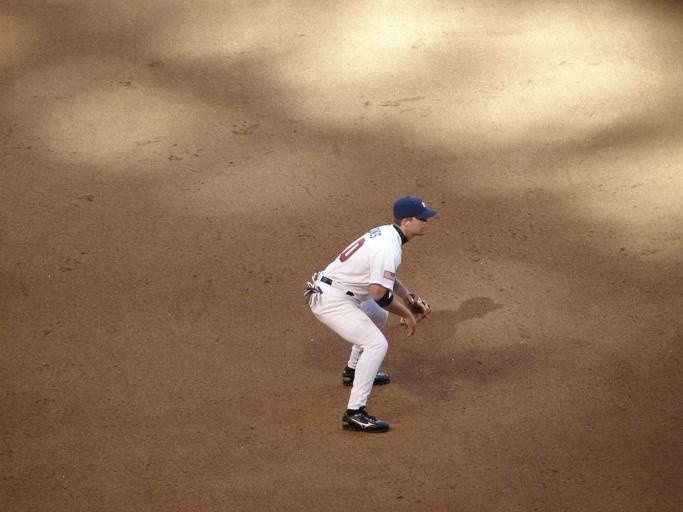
[304,271,321,307]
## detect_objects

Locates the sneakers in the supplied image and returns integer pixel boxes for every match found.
[340,405,391,434]
[339,364,392,387]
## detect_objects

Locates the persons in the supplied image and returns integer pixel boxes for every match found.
[305,197,439,432]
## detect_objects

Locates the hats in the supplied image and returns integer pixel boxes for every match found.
[393,194,438,221]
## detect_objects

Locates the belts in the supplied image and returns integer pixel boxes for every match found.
[311,272,357,298]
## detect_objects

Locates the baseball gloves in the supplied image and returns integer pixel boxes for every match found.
[400,292,431,326]
